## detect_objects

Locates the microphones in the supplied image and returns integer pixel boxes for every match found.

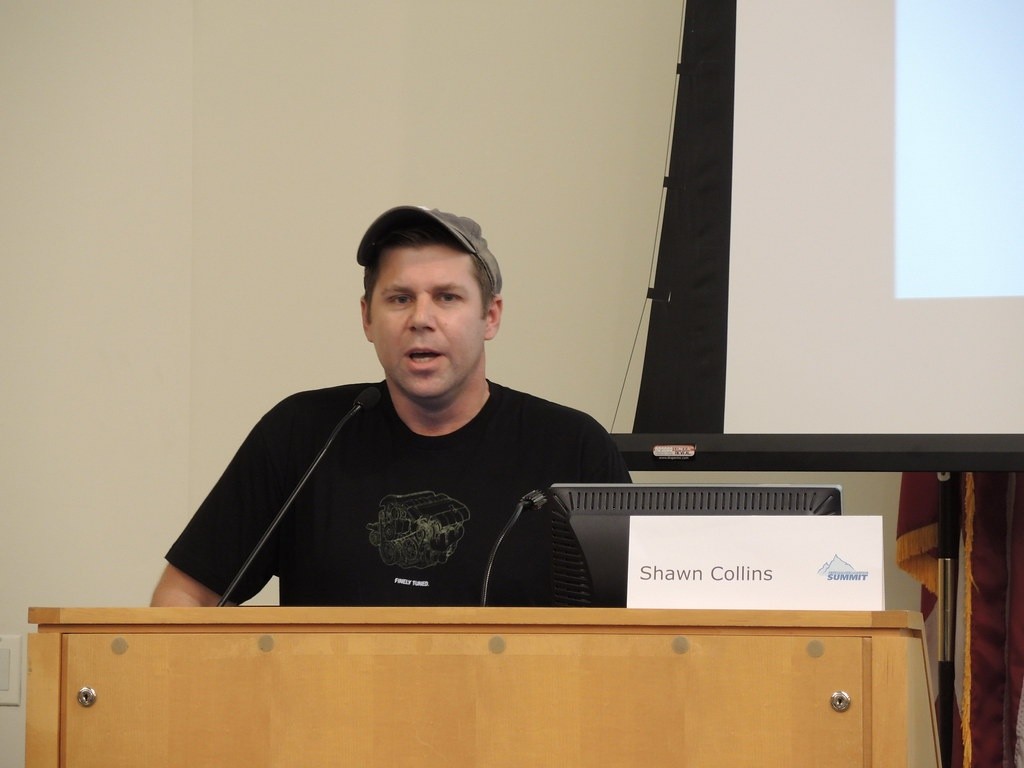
[481,488,548,606]
[216,385,390,608]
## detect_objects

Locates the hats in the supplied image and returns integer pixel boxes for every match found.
[357,205,502,294]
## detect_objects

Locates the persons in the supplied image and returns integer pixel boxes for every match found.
[148,203,633,610]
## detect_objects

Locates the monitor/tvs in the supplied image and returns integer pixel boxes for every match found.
[547,485,844,609]
